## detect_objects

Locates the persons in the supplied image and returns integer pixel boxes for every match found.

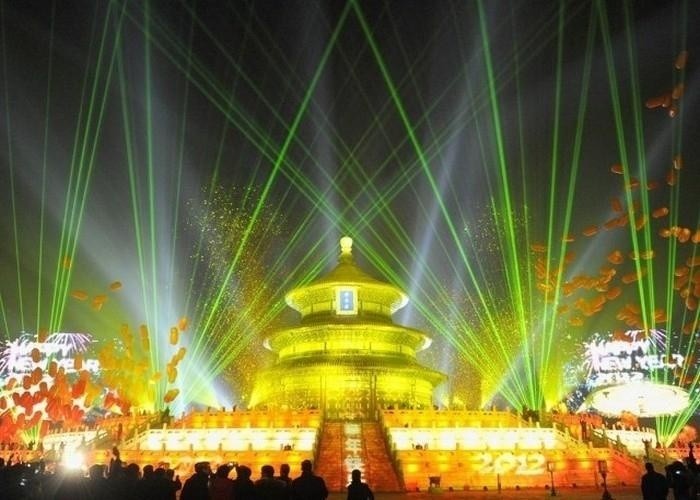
[665,460,695,500]
[179,459,329,500]
[1,451,182,500]
[345,467,374,500]
[640,462,669,500]
[579,414,588,442]
[641,438,653,459]
[1,440,67,459]
